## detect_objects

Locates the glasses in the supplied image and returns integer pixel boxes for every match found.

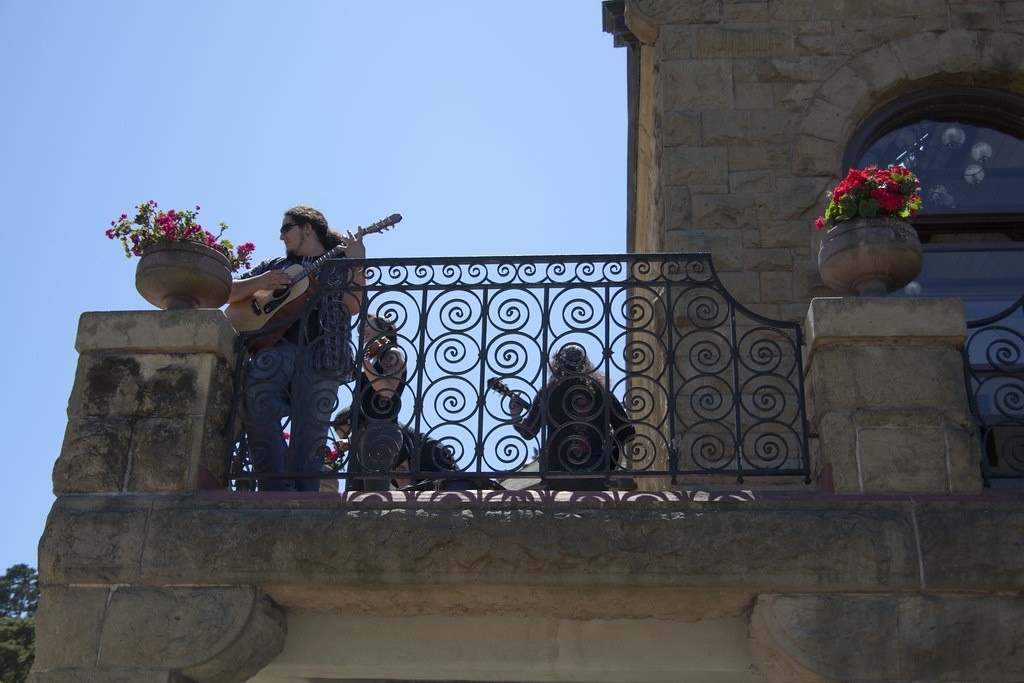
[280,223,304,234]
[340,427,352,439]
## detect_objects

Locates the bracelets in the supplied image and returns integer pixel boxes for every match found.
[356,268,363,272]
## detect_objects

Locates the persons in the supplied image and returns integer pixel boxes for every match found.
[225,206,365,492]
[334,406,460,492]
[332,313,408,492]
[510,347,637,492]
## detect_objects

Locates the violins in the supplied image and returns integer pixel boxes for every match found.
[364,336,391,359]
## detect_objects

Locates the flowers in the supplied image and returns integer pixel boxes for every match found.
[284,431,345,470]
[105,200,255,273]
[816,163,923,224]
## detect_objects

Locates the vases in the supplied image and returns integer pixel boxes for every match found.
[818,217,923,297]
[319,479,340,492]
[135,242,232,309]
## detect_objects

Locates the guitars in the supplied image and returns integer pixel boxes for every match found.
[225,213,402,351]
[487,376,533,412]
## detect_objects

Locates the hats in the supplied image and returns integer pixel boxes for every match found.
[368,313,396,330]
